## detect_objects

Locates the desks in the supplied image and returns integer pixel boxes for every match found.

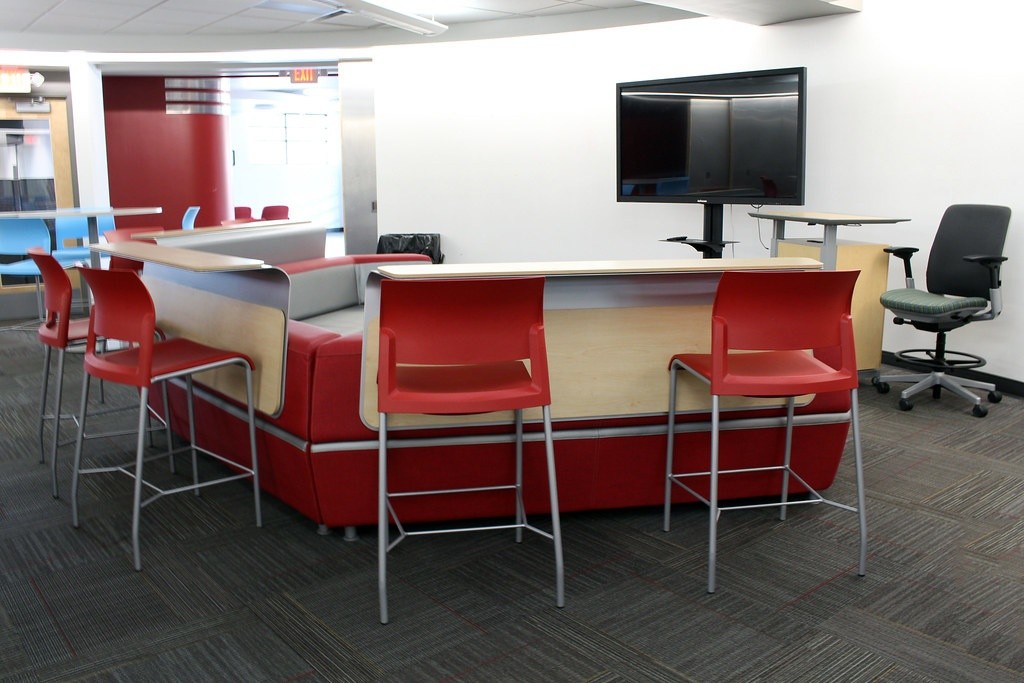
[360,256,825,432]
[88,221,328,418]
[747,210,912,387]
[0,208,162,221]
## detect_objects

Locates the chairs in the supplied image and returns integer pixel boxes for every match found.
[378,275,564,624]
[235,206,289,221]
[0,206,116,333]
[27,247,177,501]
[98,225,167,405]
[871,205,1011,417]
[663,269,866,594]
[73,266,261,572]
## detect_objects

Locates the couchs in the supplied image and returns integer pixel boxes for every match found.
[140,254,850,542]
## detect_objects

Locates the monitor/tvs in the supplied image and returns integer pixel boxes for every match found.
[616,67,809,206]
